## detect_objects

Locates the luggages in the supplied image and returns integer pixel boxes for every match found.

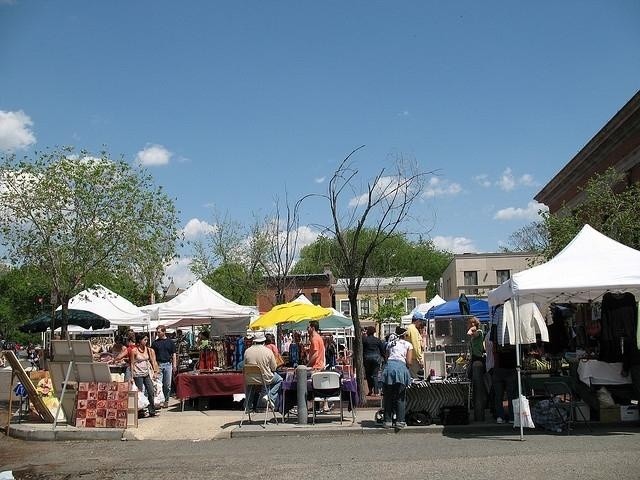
[435,404,470,426]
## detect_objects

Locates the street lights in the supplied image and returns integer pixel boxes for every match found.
[387,253,396,274]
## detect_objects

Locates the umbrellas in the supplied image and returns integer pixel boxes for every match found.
[19,309,110,334]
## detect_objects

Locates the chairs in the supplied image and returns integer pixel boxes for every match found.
[311,372,342,426]
[543,382,592,436]
[239,365,278,428]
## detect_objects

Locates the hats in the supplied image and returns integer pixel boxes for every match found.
[252,330,267,342]
[412,312,427,321]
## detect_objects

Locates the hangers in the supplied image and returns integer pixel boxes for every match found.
[609,288,628,296]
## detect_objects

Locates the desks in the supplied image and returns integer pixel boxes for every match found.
[109,364,127,383]
[516,370,584,431]
[173,369,247,426]
[403,378,472,425]
[282,380,356,426]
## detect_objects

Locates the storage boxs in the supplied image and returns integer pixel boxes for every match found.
[26,371,47,380]
[575,401,590,421]
[423,351,447,382]
[600,405,640,424]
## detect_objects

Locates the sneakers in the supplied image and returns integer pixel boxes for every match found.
[262,394,276,409]
[496,416,506,424]
[508,419,514,424]
[244,406,254,414]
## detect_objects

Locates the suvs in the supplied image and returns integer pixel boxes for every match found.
[15,344,24,351]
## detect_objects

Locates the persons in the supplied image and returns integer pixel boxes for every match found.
[14,341,33,352]
[242,321,335,415]
[489,323,518,424]
[109,325,191,418]
[363,312,427,430]
[466,316,486,422]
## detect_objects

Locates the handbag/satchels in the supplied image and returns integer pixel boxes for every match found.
[405,410,433,426]
